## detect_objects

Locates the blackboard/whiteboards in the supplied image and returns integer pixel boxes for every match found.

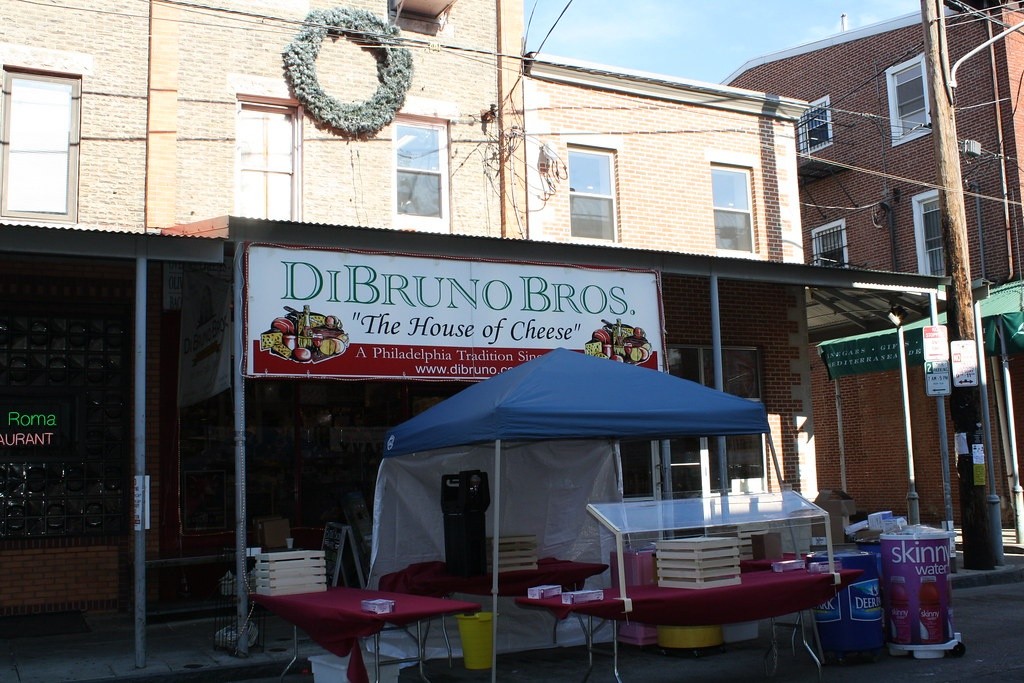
[320,521,347,586]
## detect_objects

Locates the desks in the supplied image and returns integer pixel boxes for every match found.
[514,567,865,683]
[248,586,481,683]
[379,557,609,668]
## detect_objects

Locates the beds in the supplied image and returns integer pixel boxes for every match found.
[811,500,907,546]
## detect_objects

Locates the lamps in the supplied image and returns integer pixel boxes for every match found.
[888,313,901,327]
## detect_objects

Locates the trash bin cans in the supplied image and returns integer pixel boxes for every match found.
[457,611,499,669]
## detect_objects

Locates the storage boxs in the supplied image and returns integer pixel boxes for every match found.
[486,535,540,574]
[656,535,741,589]
[772,560,805,573]
[252,516,283,547]
[719,620,760,644]
[616,619,658,646]
[810,561,842,573]
[309,651,401,683]
[254,550,327,598]
[256,517,291,547]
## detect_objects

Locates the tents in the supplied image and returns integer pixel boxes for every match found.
[369,346,829,683]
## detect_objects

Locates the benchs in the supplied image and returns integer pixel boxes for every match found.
[123,546,303,614]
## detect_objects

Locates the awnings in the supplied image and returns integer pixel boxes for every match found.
[0,217,226,266]
[160,215,952,294]
[814,276,1024,374]
